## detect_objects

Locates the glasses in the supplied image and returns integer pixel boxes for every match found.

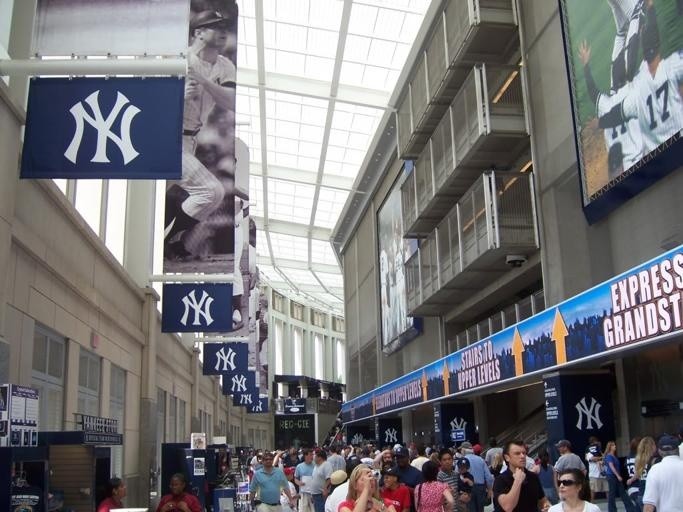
[556,478,580,487]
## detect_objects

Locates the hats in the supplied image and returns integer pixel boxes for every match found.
[457,457,471,469]
[329,469,347,486]
[457,442,483,455]
[393,446,411,457]
[554,438,573,448]
[381,467,399,478]
[657,434,681,452]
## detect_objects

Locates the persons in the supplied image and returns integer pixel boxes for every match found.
[214,429,683,512]
[575,0,683,180]
[379,213,413,348]
[232,189,268,387]
[157,473,203,512]
[166,9,236,262]
[97,477,127,511]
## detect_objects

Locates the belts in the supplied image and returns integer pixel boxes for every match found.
[262,501,281,506]
